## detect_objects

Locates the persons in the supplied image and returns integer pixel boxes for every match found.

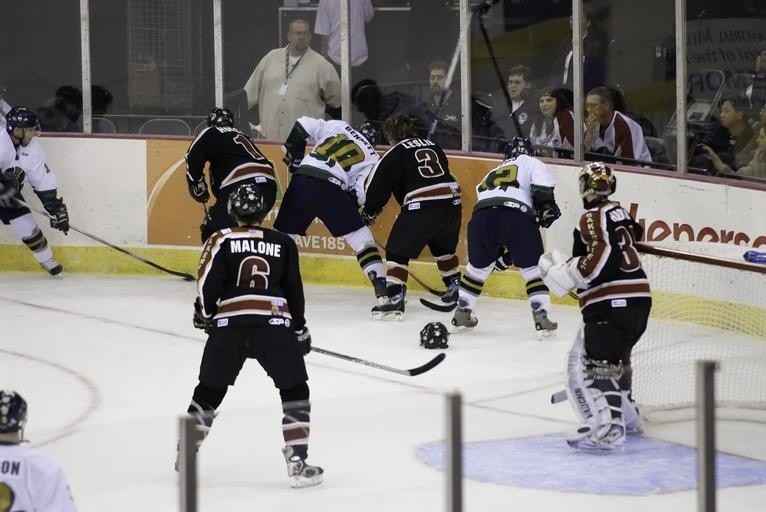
[0,391,72,511]
[274,115,388,298]
[36,84,82,128]
[353,50,765,181]
[546,162,652,449]
[185,107,277,244]
[244,19,342,142]
[90,84,112,121]
[361,113,462,311]
[451,136,561,330]
[560,6,609,94]
[1,108,69,273]
[175,184,324,488]
[310,0,375,86]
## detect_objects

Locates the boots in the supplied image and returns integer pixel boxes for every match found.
[39,257,63,275]
[282,445,324,478]
[441,271,478,327]
[530,299,558,331]
[367,270,406,313]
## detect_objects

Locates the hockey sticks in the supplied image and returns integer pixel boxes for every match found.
[374,234,452,298]
[420,298,458,314]
[13,196,194,283]
[309,345,445,380]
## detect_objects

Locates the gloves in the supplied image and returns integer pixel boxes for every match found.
[538,250,591,297]
[532,195,561,228]
[296,326,312,356]
[361,208,378,226]
[186,174,210,203]
[193,296,215,329]
[285,151,304,173]
[44,197,70,235]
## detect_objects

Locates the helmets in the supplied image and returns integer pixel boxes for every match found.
[355,121,383,148]
[0,389,27,433]
[206,108,234,127]
[504,136,534,160]
[5,106,38,135]
[350,79,381,115]
[227,183,267,220]
[578,161,616,210]
[56,86,82,118]
[90,85,112,115]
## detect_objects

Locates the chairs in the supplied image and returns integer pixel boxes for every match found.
[643,135,667,164]
[193,119,211,137]
[137,119,191,137]
[80,118,117,136]
